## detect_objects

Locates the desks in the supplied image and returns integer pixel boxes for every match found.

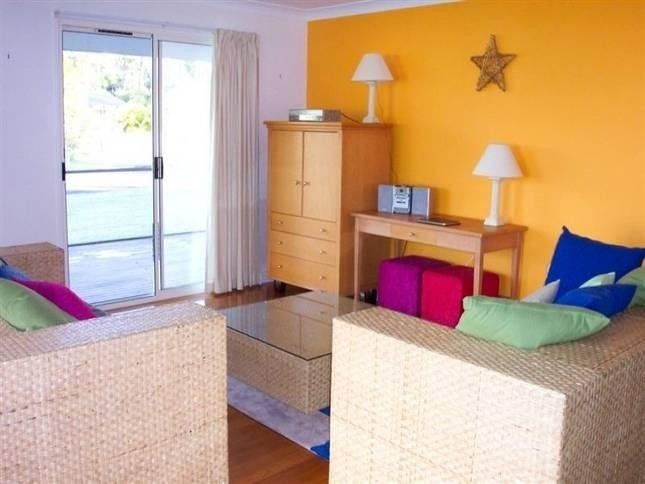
[351,209,528,307]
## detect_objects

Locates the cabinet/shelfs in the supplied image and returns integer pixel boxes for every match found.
[262,119,393,298]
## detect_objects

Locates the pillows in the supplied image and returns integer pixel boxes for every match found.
[555,283,637,317]
[0,264,30,281]
[544,225,645,294]
[11,276,95,320]
[0,276,80,332]
[578,272,616,288]
[521,279,561,304]
[455,294,610,350]
[616,266,645,307]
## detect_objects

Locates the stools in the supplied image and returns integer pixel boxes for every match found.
[420,264,499,328]
[375,255,450,316]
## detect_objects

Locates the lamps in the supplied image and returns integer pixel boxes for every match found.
[350,52,394,123]
[471,143,523,228]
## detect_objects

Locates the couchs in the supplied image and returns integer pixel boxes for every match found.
[327,305,645,484]
[0,241,231,484]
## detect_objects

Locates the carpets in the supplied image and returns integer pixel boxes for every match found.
[227,374,330,462]
